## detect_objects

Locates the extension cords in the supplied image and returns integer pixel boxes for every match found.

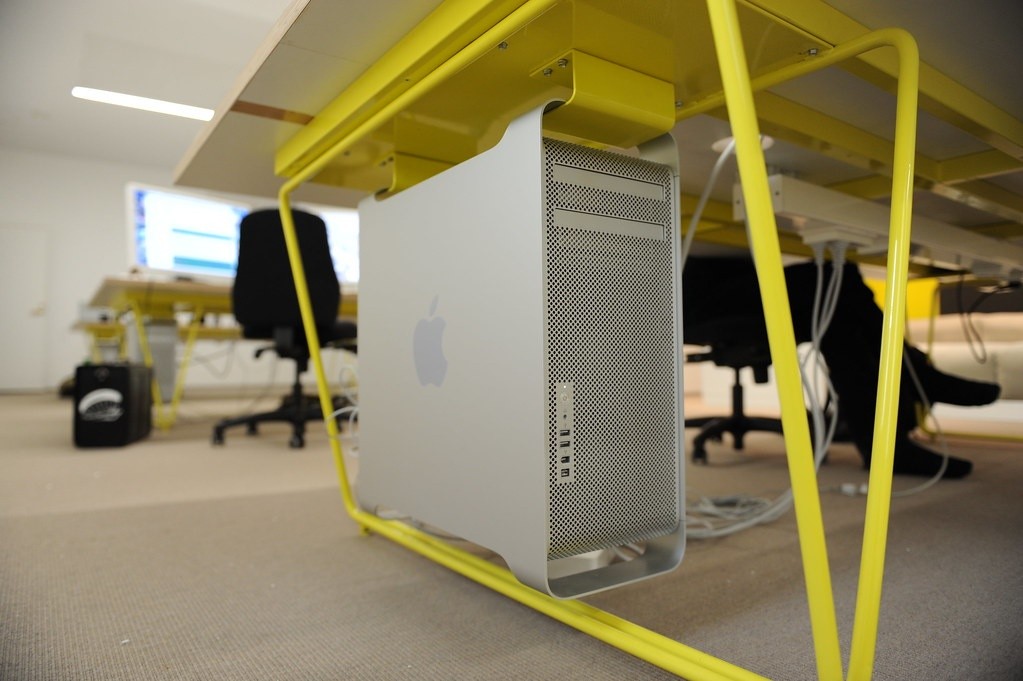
[733,175,1023,277]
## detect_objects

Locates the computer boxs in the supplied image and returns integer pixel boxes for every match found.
[356,96,687,599]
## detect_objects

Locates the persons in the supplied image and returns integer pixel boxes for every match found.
[687,256,1001,481]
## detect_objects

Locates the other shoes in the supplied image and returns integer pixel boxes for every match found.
[864,440,973,479]
[915,367,1000,406]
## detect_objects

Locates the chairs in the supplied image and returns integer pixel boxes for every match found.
[684,313,833,467]
[211,206,357,448]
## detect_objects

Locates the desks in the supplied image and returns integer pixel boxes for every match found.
[87,277,356,434]
[73,320,249,366]
[174,0,1023,681]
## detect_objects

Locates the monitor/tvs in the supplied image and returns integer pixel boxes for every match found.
[126,182,256,287]
